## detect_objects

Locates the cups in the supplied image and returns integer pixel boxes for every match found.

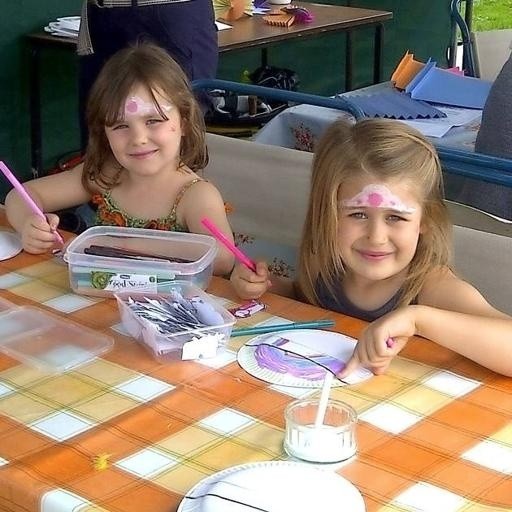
[248,93,258,116]
[283,399,360,467]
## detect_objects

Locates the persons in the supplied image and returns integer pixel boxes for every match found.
[77,1,219,152]
[4,40,235,276]
[230,113,512,379]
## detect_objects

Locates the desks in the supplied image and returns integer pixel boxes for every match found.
[0,206,512,512]
[244,77,482,155]
[25,1,393,174]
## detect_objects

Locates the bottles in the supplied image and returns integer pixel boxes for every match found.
[234,70,254,115]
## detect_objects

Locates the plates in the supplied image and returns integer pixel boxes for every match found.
[235,328,374,390]
[0,229,23,261]
[177,460,367,512]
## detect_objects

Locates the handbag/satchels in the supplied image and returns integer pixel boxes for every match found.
[203,66,302,128]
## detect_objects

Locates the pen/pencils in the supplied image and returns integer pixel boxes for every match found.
[200,216,256,273]
[0,161,64,246]
[72,245,205,300]
[231,319,336,337]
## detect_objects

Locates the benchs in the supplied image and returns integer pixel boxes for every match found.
[196,132,512,318]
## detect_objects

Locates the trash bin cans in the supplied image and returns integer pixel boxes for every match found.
[203,92,289,141]
